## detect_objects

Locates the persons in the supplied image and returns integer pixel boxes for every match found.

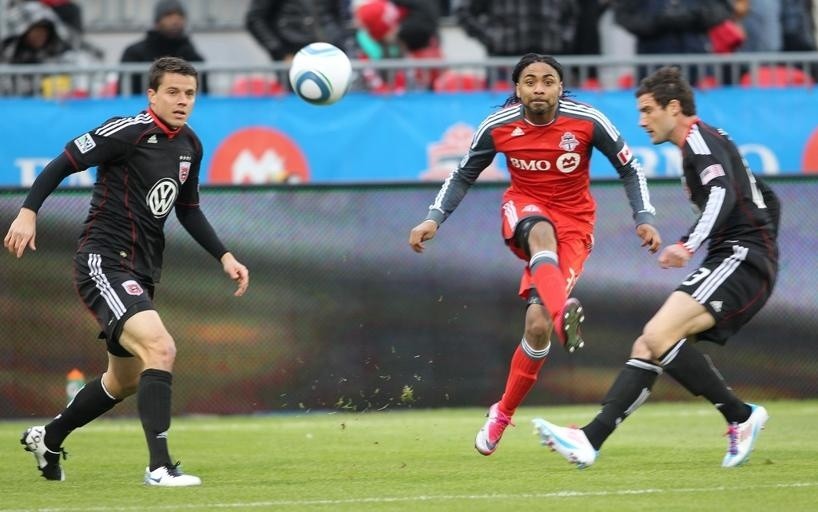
[3,57,249,487]
[408,53,661,456]
[531,67,782,474]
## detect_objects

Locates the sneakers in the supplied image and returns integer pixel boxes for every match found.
[722,403,770,468]
[554,296,584,353]
[532,417,599,468]
[476,402,511,454]
[143,465,201,487]
[21,424,65,482]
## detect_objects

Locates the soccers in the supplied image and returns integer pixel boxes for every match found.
[291,42,351,104]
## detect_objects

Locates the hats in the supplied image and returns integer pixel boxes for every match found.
[356,1,407,42]
[155,0,186,22]
